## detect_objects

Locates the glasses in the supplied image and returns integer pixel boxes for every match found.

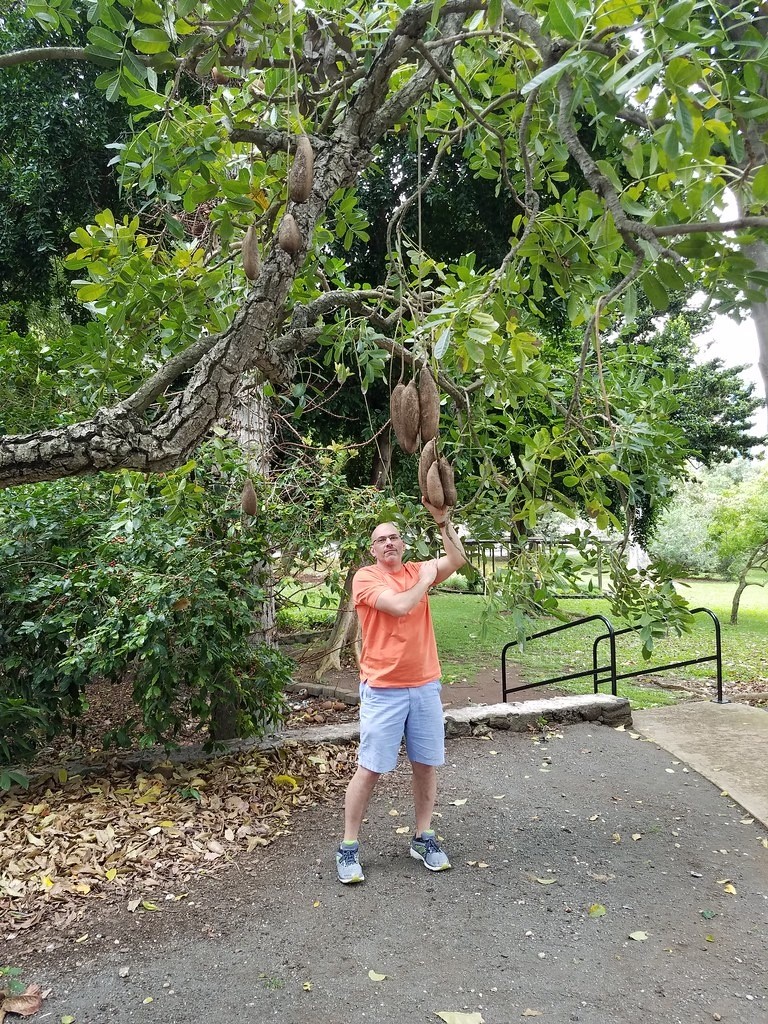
[372,535,401,546]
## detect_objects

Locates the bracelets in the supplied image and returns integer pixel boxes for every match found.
[438,521,446,528]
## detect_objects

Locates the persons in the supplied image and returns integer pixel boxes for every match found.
[336,496,466,883]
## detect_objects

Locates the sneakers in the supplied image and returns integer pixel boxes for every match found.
[410,830,451,870]
[335,840,364,883]
